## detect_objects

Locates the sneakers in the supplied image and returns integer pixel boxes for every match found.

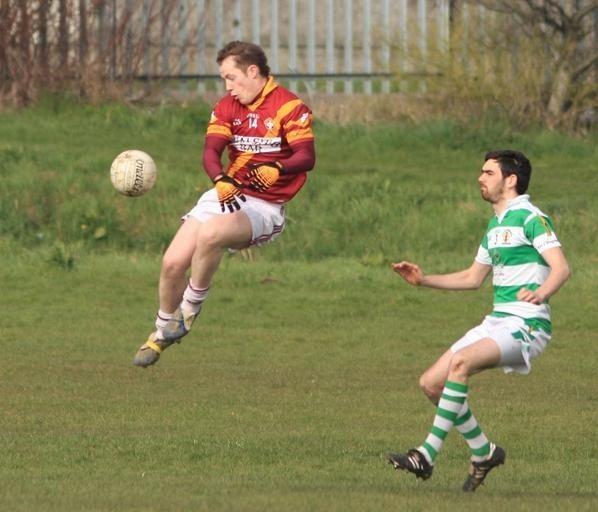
[134,330,177,369]
[161,298,204,342]
[385,446,433,482]
[461,439,506,494]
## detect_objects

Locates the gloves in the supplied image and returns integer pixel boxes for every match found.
[243,160,285,192]
[213,174,249,213]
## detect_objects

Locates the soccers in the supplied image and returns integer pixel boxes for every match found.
[110,150,156,196]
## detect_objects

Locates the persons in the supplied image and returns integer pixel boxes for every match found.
[134,41,315,367]
[389,149,571,493]
